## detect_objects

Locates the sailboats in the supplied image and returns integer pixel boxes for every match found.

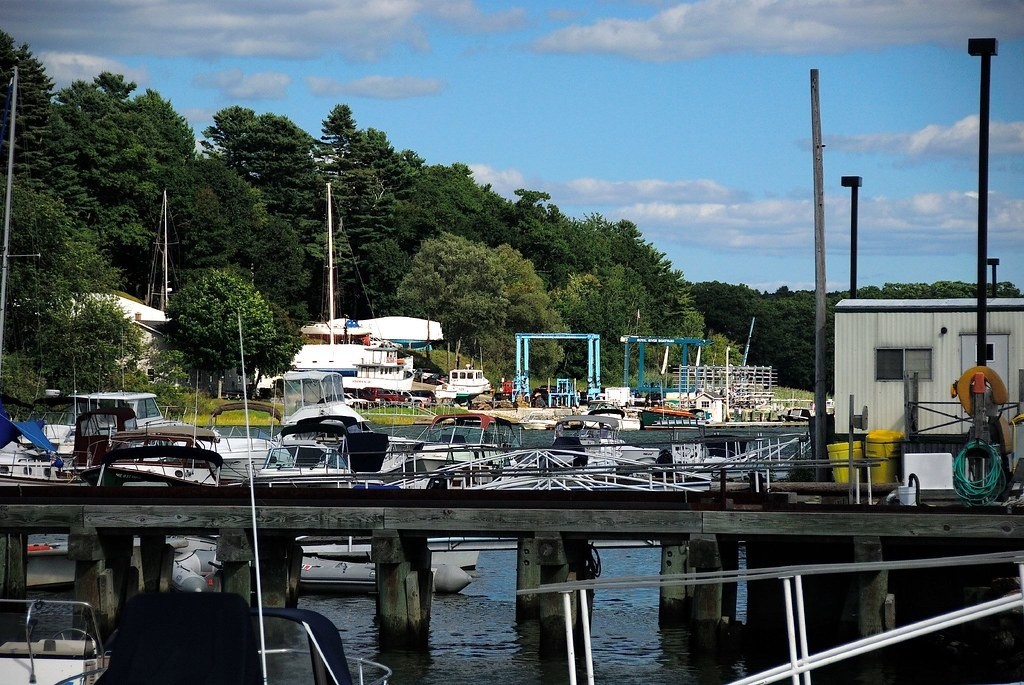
[254,183,418,391]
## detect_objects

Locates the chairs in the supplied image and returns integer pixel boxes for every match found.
[91,592,263,685]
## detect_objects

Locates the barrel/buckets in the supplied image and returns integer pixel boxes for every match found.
[865,430,905,482]
[826,441,863,483]
[898,485,917,506]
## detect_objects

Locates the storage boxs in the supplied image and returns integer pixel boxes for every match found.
[904,453,954,487]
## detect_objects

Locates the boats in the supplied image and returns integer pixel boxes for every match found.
[172,551,375,593]
[640,406,714,429]
[434,369,492,403]
[519,410,558,430]
[0,371,388,491]
[777,407,811,421]
[298,316,443,349]
[540,416,660,473]
[0,591,393,685]
[401,413,525,478]
[581,402,640,431]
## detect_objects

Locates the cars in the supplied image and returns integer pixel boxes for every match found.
[396,390,430,408]
[411,390,437,407]
[413,368,434,379]
[343,393,372,409]
[358,387,408,408]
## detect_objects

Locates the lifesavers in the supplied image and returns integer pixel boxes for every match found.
[957,367,1010,416]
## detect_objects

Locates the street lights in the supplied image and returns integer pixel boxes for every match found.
[969,37,999,367]
[841,175,861,298]
